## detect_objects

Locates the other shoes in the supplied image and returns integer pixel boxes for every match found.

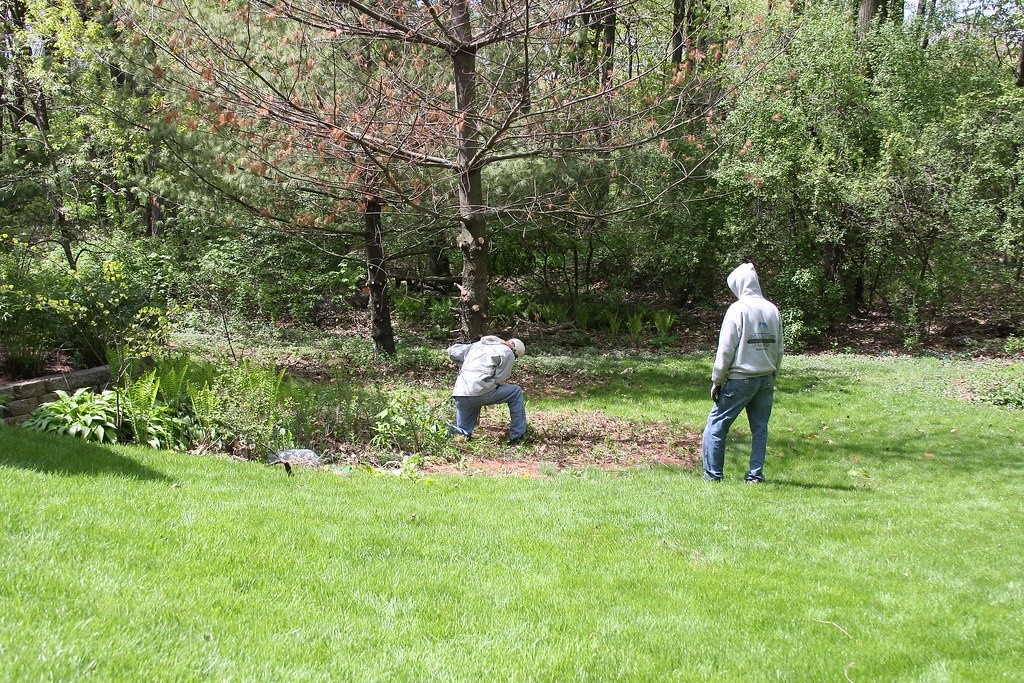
[508,434,524,445]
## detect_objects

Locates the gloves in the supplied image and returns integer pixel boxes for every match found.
[710,385,721,402]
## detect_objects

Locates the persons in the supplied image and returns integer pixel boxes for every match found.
[701,263,785,483]
[429,335,527,445]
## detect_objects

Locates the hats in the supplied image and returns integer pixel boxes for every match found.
[509,338,525,358]
